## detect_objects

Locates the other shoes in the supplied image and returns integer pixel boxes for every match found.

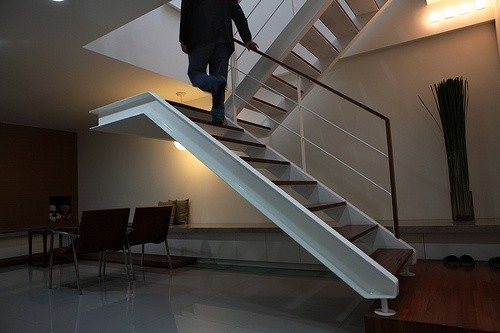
[211,78,227,106]
[213,119,224,125]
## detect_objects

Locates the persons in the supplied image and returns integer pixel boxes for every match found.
[179,0,260,126]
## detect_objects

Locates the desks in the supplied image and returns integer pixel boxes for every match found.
[28,228,79,268]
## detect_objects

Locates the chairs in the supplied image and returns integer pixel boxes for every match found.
[50,208,135,296]
[102,206,172,282]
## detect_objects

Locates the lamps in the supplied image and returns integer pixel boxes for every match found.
[174,92,186,150]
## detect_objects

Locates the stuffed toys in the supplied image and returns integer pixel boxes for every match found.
[48,204,62,222]
[59,204,71,220]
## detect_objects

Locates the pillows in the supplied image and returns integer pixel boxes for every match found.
[158,200,177,226]
[176,199,189,224]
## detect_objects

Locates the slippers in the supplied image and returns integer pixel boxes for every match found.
[459,255,475,267]
[489,256,500,268]
[443,254,461,266]
